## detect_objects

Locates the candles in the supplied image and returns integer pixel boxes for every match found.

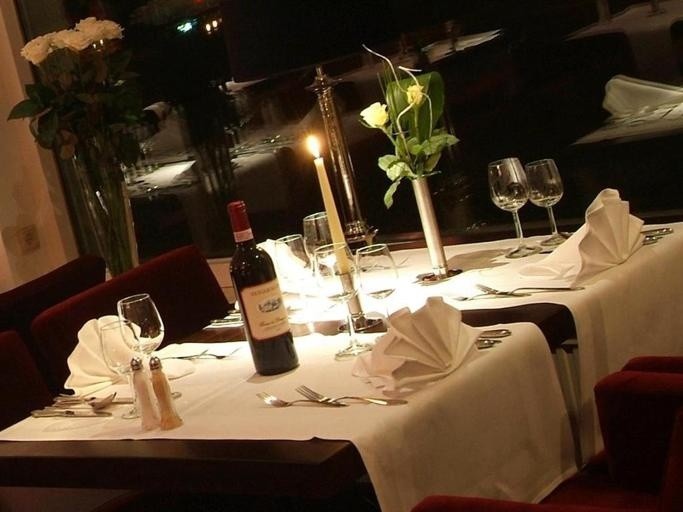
[301,134,353,273]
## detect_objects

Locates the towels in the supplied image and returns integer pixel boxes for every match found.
[601,72,683,115]
[602,73,683,125]
[517,190,643,284]
[369,298,480,388]
[67,316,198,393]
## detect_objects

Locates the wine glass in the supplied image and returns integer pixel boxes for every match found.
[101,320,139,418]
[272,232,317,335]
[525,158,573,246]
[116,292,181,400]
[303,212,338,282]
[488,156,543,259]
[357,244,401,331]
[315,241,371,361]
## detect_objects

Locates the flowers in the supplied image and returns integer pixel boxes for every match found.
[358,43,461,208]
[7,15,162,175]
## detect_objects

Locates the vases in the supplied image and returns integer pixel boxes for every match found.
[72,155,140,279]
[33,128,139,270]
[410,177,462,283]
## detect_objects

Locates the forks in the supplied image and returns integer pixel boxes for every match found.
[452,292,532,302]
[296,384,407,406]
[476,282,584,294]
[256,387,349,408]
[45,394,113,411]
[228,306,300,315]
[160,348,209,360]
[203,352,238,359]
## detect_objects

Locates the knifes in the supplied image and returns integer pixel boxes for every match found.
[31,410,112,417]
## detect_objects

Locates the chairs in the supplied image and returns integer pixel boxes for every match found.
[409,369,683,512]
[0,329,52,429]
[31,244,232,392]
[622,354,683,369]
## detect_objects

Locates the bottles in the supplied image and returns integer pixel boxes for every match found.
[129,356,185,432]
[227,200,299,377]
